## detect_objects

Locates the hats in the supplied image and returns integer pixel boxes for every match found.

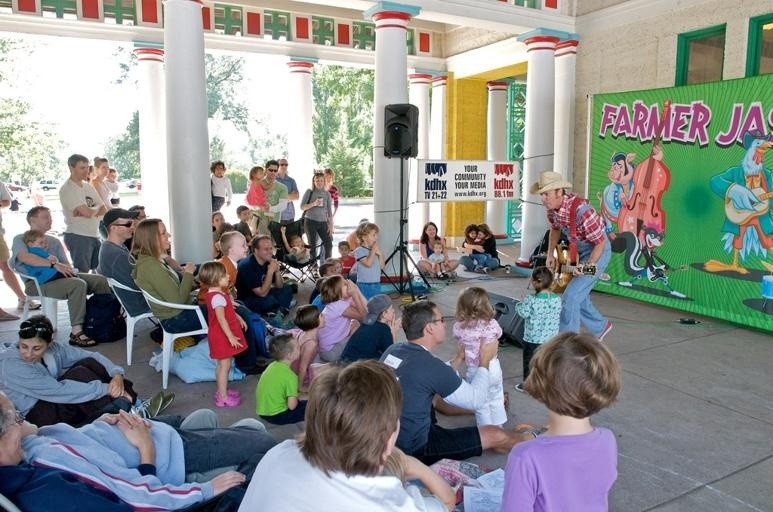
[102,208,140,227]
[361,293,393,326]
[529,170,573,195]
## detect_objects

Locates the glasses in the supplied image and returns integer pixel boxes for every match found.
[268,169,278,172]
[110,222,131,228]
[313,173,324,179]
[20,321,53,332]
[427,316,445,324]
[280,164,288,166]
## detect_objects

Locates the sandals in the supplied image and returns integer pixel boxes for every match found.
[213,396,240,407]
[511,423,549,441]
[68,331,96,347]
[212,384,240,399]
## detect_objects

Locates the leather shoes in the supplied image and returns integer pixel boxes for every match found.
[435,272,445,280]
[441,271,451,279]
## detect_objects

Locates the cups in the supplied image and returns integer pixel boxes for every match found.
[317,196,324,208]
[505,265,511,274]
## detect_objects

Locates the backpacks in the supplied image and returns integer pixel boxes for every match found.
[84,292,127,344]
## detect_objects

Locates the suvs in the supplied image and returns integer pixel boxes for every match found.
[39,180,58,191]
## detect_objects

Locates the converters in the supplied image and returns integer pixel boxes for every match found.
[680,318,697,324]
[419,295,427,300]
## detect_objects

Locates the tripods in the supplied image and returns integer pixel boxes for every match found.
[381,160,431,301]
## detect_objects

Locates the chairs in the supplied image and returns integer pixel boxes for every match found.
[269,220,328,288]
[0,493,22,512]
[105,276,155,367]
[138,288,209,389]
[6,257,66,334]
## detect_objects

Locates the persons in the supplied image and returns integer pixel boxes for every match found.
[58,152,107,275]
[529,170,613,342]
[2,158,561,511]
[499,332,622,512]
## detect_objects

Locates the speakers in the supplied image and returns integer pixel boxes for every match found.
[384,104,419,159]
[487,292,525,348]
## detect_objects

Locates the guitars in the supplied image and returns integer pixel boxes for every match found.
[725,188,772,225]
[549,245,597,293]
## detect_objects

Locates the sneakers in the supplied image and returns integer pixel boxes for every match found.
[514,383,526,393]
[135,393,174,416]
[132,391,162,418]
[473,267,490,274]
[0,306,20,320]
[17,297,41,310]
[599,320,612,341]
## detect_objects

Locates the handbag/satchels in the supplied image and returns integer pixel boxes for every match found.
[299,217,304,235]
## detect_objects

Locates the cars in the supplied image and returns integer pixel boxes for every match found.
[125,179,138,190]
[4,183,26,193]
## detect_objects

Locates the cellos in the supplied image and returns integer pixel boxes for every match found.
[618,100,671,248]
